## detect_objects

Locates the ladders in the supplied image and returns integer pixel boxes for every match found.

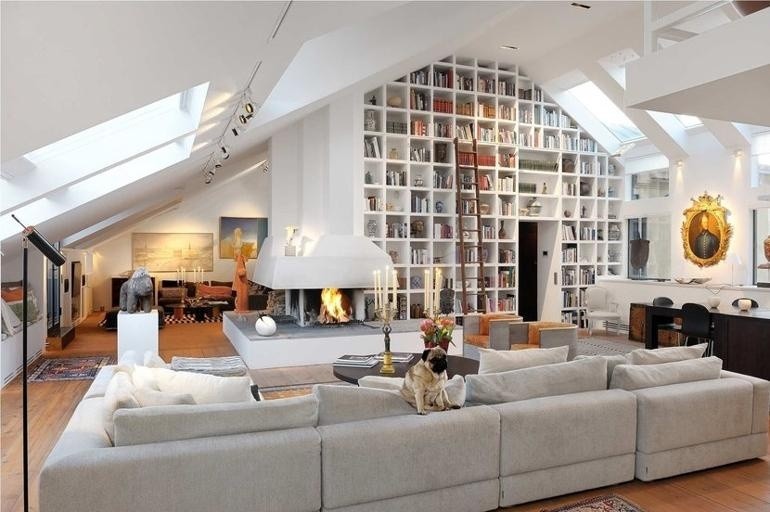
[453,137,487,315]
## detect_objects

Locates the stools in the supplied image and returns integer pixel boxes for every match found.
[560,306,587,328]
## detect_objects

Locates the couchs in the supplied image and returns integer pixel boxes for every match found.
[38,392,322,512]
[314,403,501,512]
[632,369,770,482]
[508,321,579,362]
[462,313,523,362]
[486,387,638,508]
[81,366,263,402]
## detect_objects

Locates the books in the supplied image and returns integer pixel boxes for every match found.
[498,177,514,193]
[456,102,475,115]
[410,71,428,85]
[535,89,544,103]
[581,268,594,286]
[499,268,515,288]
[458,248,475,262]
[580,310,588,329]
[374,350,414,363]
[477,124,494,141]
[369,196,381,211]
[479,173,494,190]
[434,100,454,113]
[565,312,578,326]
[563,268,577,285]
[564,292,579,307]
[479,155,496,166]
[411,90,430,110]
[434,71,450,87]
[518,132,533,147]
[499,198,513,215]
[434,171,453,189]
[387,222,407,238]
[411,195,429,213]
[364,136,382,158]
[519,88,532,100]
[563,248,576,263]
[561,225,577,240]
[498,249,515,263]
[434,223,454,238]
[520,159,554,171]
[499,105,516,120]
[534,108,541,124]
[478,103,496,117]
[459,173,473,190]
[434,121,453,138]
[535,130,541,147]
[333,354,378,368]
[580,137,595,152]
[498,80,516,96]
[457,151,476,165]
[387,120,407,134]
[412,120,427,136]
[411,248,429,264]
[581,160,595,175]
[518,109,533,124]
[580,227,596,240]
[499,295,516,311]
[499,128,517,144]
[561,114,572,128]
[544,134,560,148]
[481,225,496,239]
[563,133,578,150]
[477,78,495,93]
[411,147,430,161]
[580,289,592,309]
[456,198,474,214]
[478,294,496,313]
[390,170,407,186]
[519,182,537,193]
[457,123,473,139]
[457,74,473,91]
[563,182,578,196]
[544,110,559,127]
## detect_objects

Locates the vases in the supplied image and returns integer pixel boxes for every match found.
[423,335,450,354]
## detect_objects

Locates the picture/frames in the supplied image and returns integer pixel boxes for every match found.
[677,190,735,270]
[219,216,268,259]
[131,232,213,273]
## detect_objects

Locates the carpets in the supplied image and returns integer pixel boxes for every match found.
[27,356,118,384]
[97,313,223,328]
[539,492,645,512]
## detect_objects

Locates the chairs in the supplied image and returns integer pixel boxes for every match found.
[584,285,621,338]
[681,302,716,358]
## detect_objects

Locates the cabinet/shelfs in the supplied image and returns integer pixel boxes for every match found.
[363,55,632,329]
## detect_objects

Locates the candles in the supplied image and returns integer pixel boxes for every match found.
[376,270,382,309]
[176,268,179,283]
[384,264,389,304]
[373,270,378,310]
[193,268,196,283]
[392,269,397,309]
[180,266,186,283]
[421,267,443,313]
[201,268,204,283]
[197,267,201,283]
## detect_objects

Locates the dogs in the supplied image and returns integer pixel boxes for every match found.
[399,345,461,416]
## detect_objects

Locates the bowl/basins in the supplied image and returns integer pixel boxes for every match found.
[738,299,751,310]
[708,297,720,308]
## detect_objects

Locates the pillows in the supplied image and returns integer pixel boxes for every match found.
[312,375,417,427]
[103,349,257,446]
[464,345,627,408]
[112,394,319,447]
[609,356,723,391]
[629,343,708,365]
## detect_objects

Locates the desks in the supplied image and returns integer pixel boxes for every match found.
[159,296,229,319]
[628,300,770,381]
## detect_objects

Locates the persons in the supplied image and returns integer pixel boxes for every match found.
[232,227,248,313]
[693,213,718,256]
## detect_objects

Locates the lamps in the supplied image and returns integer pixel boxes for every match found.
[214,162,222,168]
[238,115,247,124]
[284,226,299,257]
[245,101,257,114]
[209,170,215,176]
[205,179,211,184]
[222,152,230,160]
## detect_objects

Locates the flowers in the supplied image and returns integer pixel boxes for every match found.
[418,305,456,347]
